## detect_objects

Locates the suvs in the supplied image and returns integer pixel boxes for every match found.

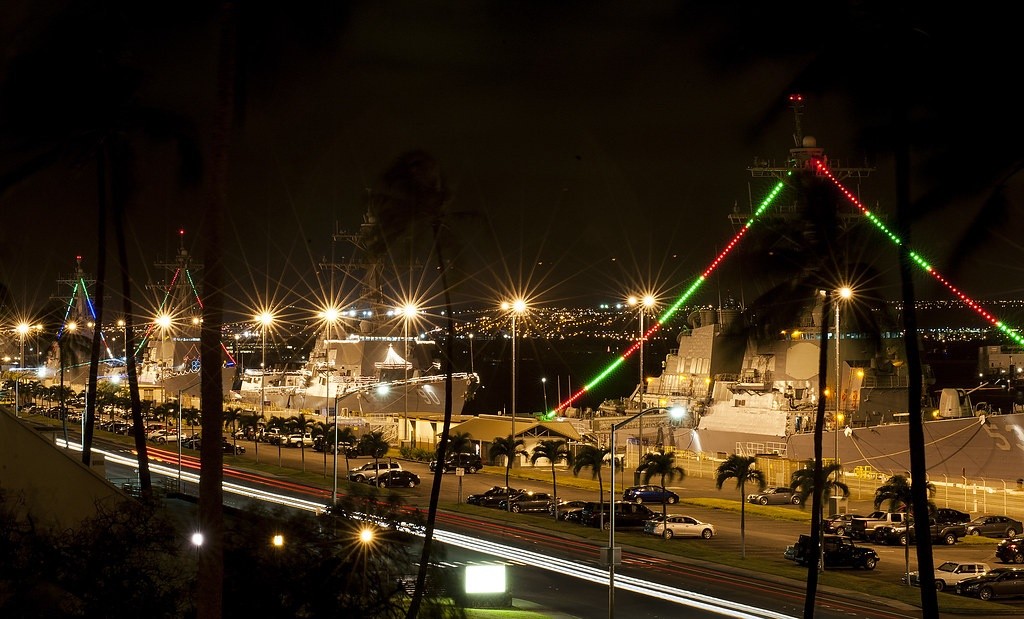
[783,533,880,571]
[346,461,402,483]
[429,451,483,474]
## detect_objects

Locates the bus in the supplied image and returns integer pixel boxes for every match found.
[934,386,1015,415]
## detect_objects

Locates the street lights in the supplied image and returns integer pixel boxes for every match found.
[607,395,688,619]
[628,292,657,470]
[834,288,853,483]
[10,287,529,460]
[358,527,376,619]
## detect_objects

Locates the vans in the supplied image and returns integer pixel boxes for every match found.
[584,502,654,530]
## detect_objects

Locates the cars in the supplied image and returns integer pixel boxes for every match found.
[368,470,421,488]
[902,559,1024,602]
[467,486,587,521]
[822,507,1024,563]
[644,514,720,537]
[624,485,679,504]
[0,384,356,455]
[747,488,802,506]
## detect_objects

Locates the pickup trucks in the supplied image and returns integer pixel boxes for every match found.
[851,511,912,531]
